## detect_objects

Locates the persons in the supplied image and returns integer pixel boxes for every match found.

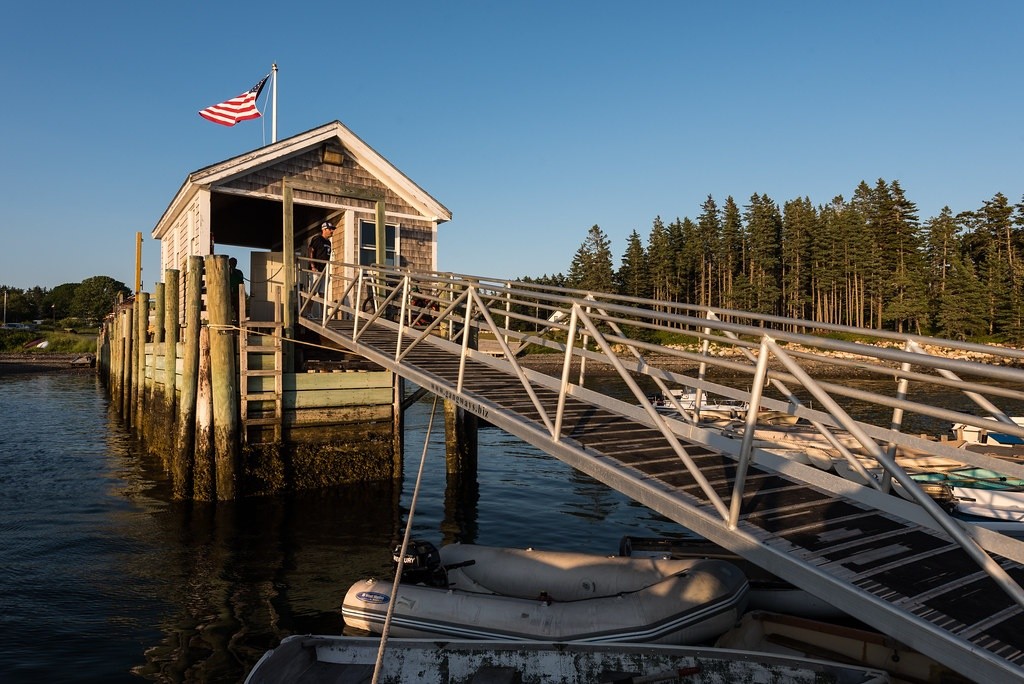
[229,258,244,322]
[303,223,336,319]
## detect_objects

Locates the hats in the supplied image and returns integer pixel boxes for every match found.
[322,222,336,230]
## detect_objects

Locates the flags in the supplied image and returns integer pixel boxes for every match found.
[199,73,269,126]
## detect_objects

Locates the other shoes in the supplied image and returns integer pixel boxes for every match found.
[307,313,317,320]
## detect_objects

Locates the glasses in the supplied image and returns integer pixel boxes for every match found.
[326,226,335,230]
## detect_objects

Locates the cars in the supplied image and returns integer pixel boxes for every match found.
[2,323,33,332]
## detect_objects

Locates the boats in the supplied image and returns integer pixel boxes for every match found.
[888,415,1024,521]
[635,388,751,414]
[247,634,891,684]
[713,608,967,684]
[341,541,753,645]
